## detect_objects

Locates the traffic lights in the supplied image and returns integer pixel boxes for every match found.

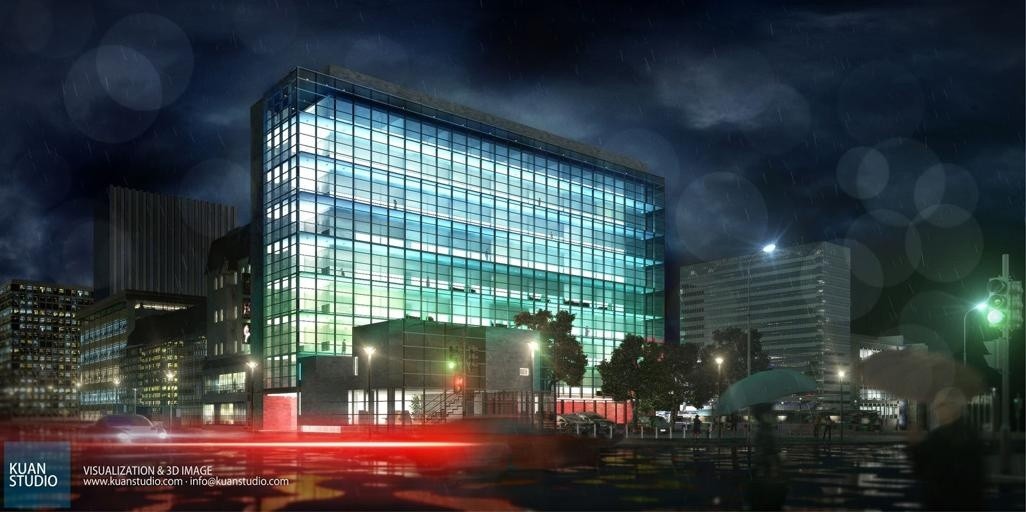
[988,275,1013,330]
[449,353,459,371]
[453,374,464,396]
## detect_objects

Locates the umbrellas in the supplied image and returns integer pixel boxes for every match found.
[715,362,821,412]
[857,349,993,400]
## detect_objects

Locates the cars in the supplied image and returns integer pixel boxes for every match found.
[532,408,713,441]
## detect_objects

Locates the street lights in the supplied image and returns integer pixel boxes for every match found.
[363,343,380,437]
[715,355,725,438]
[245,359,259,433]
[745,239,780,382]
[836,370,846,440]
[525,338,540,430]
[23,375,122,417]
[164,370,178,424]
[962,300,989,367]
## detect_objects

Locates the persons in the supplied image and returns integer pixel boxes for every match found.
[746,404,788,510]
[823,415,833,440]
[694,415,702,437]
[910,389,976,512]
[732,412,739,431]
[814,415,822,437]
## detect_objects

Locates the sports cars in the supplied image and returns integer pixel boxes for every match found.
[85,412,171,450]
